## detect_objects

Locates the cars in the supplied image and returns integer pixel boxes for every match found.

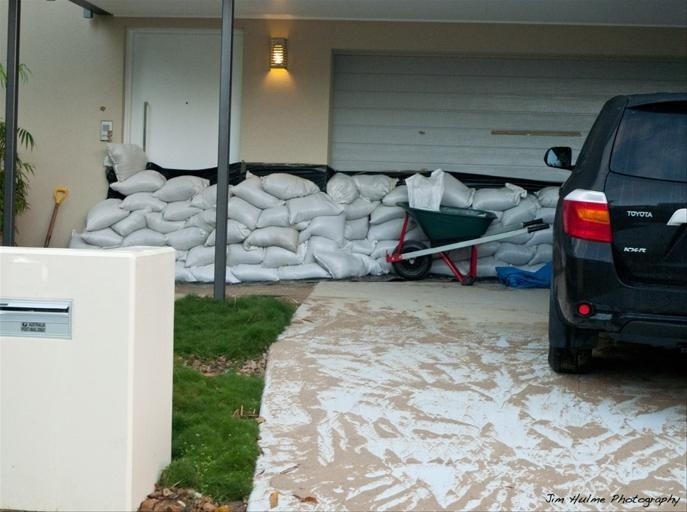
[543,92,687,374]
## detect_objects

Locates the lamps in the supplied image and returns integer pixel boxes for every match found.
[269,36,288,69]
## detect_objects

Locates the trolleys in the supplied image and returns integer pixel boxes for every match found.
[386,201,550,286]
